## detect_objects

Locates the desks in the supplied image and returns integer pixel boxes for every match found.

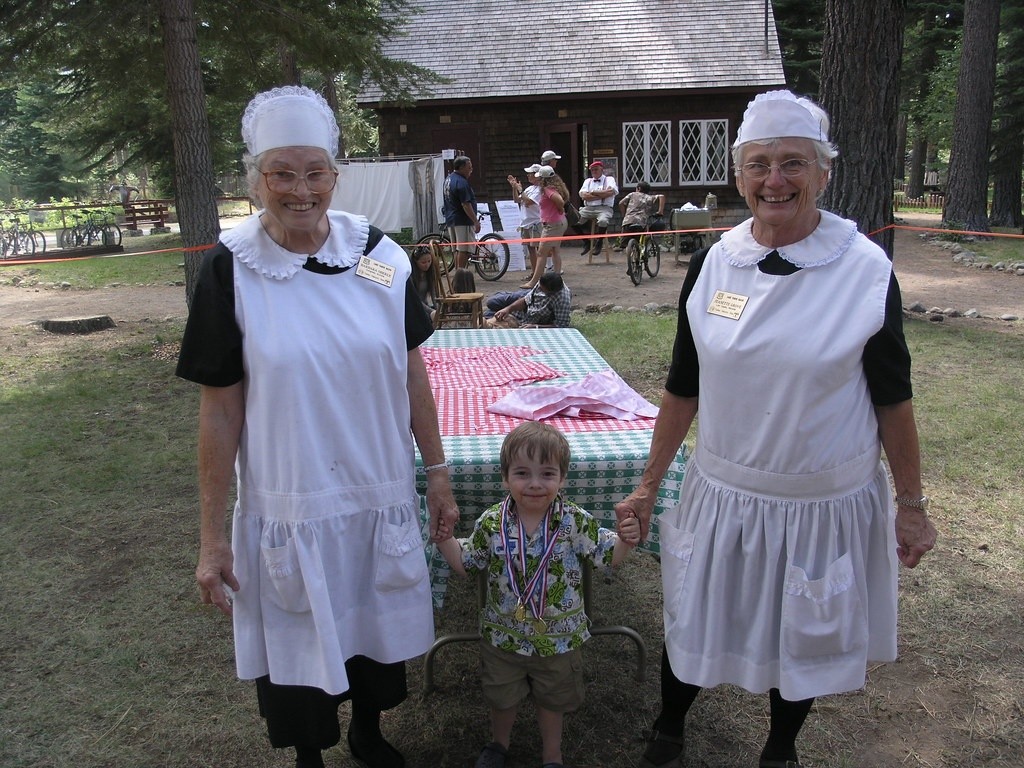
[402,327,687,696]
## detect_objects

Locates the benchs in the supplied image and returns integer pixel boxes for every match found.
[120,205,170,230]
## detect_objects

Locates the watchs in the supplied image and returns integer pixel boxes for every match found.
[422,458,453,472]
[895,495,929,510]
[591,189,594,195]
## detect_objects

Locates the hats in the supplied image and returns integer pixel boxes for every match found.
[534,165,555,178]
[524,164,541,173]
[542,150,561,160]
[589,161,603,169]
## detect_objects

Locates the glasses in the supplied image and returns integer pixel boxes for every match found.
[736,157,819,180]
[252,163,339,194]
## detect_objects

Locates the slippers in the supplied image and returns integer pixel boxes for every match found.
[475,742,508,768]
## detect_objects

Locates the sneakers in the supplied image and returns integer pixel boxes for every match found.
[521,273,533,281]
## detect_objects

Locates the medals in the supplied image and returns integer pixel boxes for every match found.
[533,616,548,635]
[515,602,526,622]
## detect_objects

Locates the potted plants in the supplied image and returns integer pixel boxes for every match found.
[99,202,116,246]
[51,196,73,247]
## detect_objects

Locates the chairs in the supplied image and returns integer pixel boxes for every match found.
[429,240,485,328]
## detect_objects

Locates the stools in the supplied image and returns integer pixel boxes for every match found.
[588,216,612,266]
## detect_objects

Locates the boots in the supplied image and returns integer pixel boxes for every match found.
[592,226,607,255]
[573,227,591,256]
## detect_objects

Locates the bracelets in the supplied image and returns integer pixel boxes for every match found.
[535,324,539,329]
[519,188,525,194]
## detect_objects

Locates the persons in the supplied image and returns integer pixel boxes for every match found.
[411,245,439,329]
[614,90,937,768]
[507,150,569,288]
[483,272,572,328]
[572,159,619,256]
[619,182,665,275]
[443,156,480,271]
[177,86,459,768]
[431,422,641,768]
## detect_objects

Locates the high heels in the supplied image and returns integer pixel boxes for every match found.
[520,281,538,289]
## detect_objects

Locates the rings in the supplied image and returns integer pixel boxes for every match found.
[454,521,457,526]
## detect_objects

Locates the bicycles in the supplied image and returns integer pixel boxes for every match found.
[0,218,48,261]
[412,211,510,281]
[626,213,662,285]
[61,209,122,249]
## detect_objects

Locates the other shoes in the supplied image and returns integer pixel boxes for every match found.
[640,719,685,768]
[759,750,803,768]
[347,719,405,768]
